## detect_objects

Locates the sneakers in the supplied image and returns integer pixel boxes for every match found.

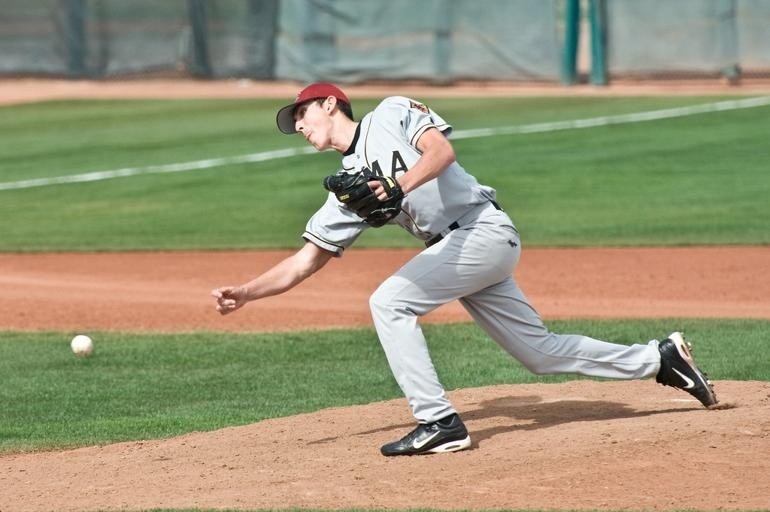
[657,331,718,409]
[380,414,471,455]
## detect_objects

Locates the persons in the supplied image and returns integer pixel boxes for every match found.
[211,82,720,455]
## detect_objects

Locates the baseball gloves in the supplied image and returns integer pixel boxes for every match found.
[323,166,404,227]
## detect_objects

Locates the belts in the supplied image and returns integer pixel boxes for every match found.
[425,199,501,249]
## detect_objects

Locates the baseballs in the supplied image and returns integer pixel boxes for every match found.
[71,335,94,357]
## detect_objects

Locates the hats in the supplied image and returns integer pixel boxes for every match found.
[276,83,350,135]
